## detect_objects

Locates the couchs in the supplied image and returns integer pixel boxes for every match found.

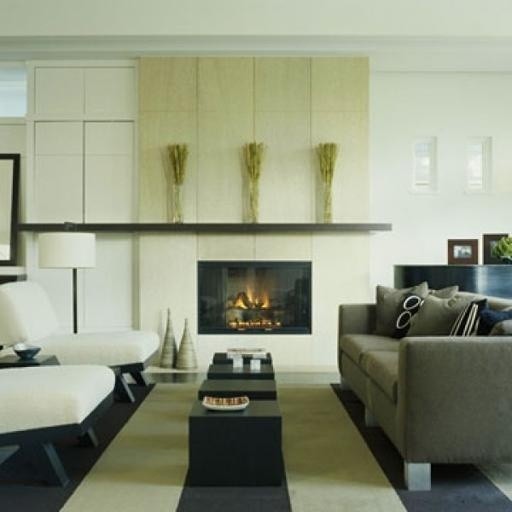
[339,282,512,495]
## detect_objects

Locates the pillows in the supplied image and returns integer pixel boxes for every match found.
[430,284,459,297]
[408,296,463,335]
[374,281,429,332]
[490,318,511,336]
[387,293,424,335]
[476,308,511,334]
[448,298,488,336]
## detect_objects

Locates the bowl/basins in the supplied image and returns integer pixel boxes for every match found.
[15,347,42,361]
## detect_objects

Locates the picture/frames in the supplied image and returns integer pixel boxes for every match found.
[1,155,21,266]
[483,235,507,263]
[445,240,481,265]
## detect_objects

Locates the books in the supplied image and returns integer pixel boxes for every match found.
[226,348,267,360]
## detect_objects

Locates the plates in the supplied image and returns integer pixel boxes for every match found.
[202,395,250,411]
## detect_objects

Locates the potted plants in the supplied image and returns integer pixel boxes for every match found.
[316,138,338,226]
[168,142,189,221]
[242,140,266,225]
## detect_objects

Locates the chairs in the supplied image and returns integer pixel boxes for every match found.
[1,361,117,490]
[1,280,162,403]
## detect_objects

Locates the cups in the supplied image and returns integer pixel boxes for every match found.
[233,356,261,371]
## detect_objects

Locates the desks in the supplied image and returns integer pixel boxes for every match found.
[2,346,63,368]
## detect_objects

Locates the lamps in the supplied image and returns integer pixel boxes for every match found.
[37,229,97,334]
